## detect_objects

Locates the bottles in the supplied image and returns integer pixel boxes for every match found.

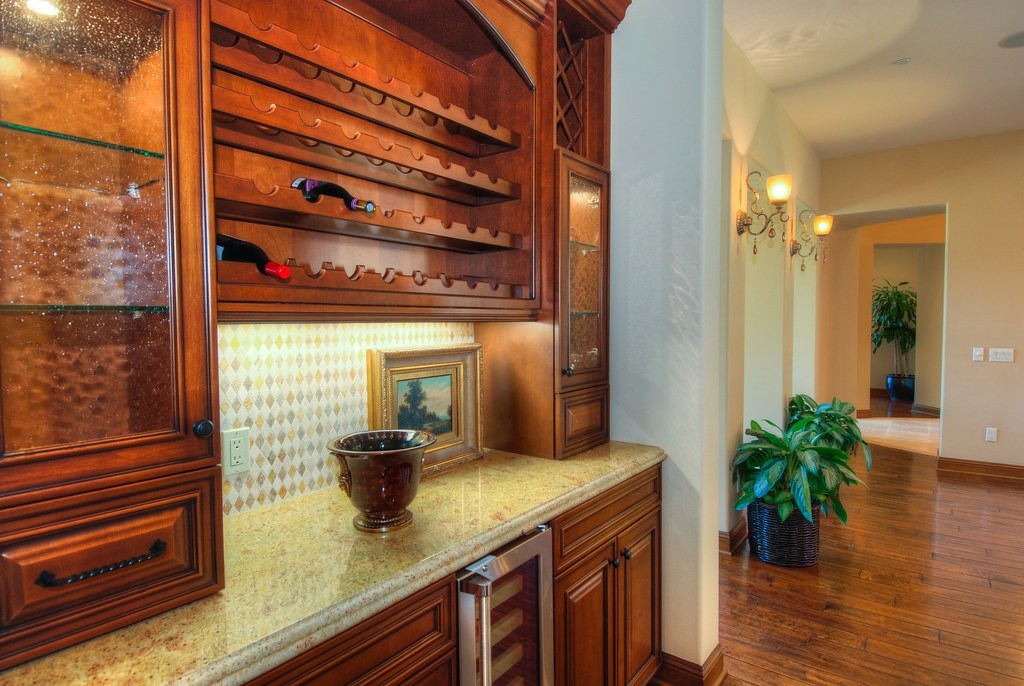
[216,233,293,281]
[290,176,375,216]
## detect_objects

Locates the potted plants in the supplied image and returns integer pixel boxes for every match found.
[729,394,871,566]
[872,277,917,401]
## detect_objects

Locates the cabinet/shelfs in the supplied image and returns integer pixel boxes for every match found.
[0,0,666,686]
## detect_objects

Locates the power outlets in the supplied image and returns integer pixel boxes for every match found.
[220,427,250,477]
[986,428,997,441]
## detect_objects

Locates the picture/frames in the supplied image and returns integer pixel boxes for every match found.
[366,343,483,482]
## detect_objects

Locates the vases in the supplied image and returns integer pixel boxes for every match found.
[326,428,437,533]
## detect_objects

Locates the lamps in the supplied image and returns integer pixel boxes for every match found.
[790,210,834,271]
[736,169,793,254]
